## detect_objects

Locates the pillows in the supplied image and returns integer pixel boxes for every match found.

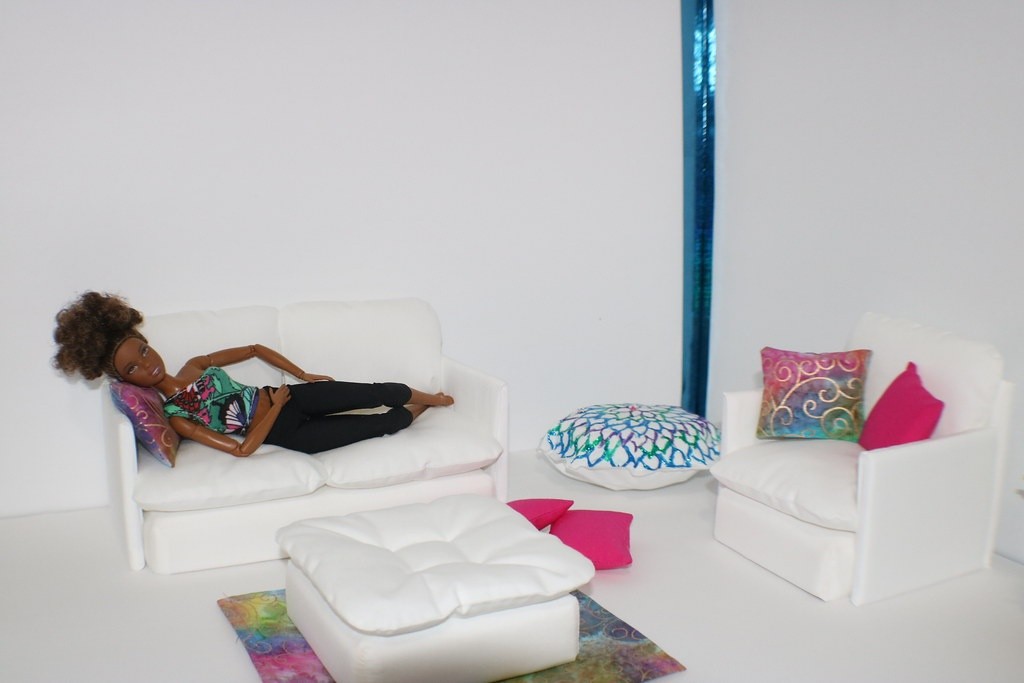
[538,403,726,491]
[548,510,635,570]
[752,346,872,442]
[504,494,576,533]
[858,360,946,459]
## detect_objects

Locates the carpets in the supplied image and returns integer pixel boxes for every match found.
[215,574,689,683]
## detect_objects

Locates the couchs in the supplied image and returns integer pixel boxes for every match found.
[712,332,1016,605]
[102,294,513,578]
[275,490,597,683]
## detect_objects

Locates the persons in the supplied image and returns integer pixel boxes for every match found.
[51,290,455,456]
